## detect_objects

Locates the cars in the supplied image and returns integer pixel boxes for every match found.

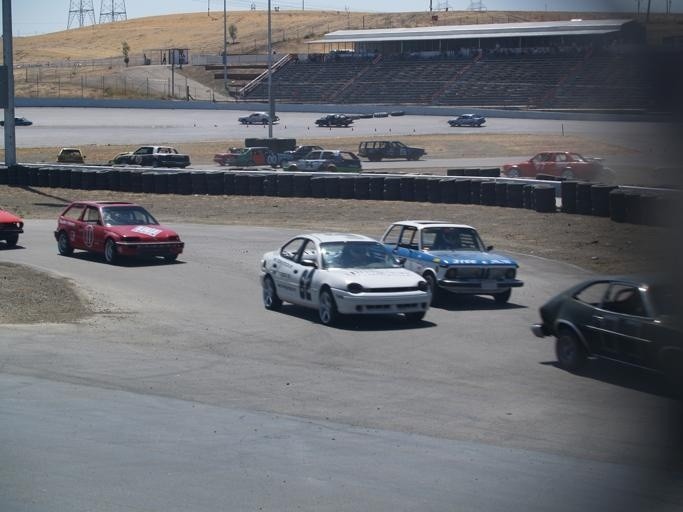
[445,110,488,126]
[0,207,26,250]
[531,277,682,375]
[380,220,524,304]
[239,109,281,126]
[53,199,186,265]
[0,113,31,127]
[256,232,435,324]
[314,112,350,127]
